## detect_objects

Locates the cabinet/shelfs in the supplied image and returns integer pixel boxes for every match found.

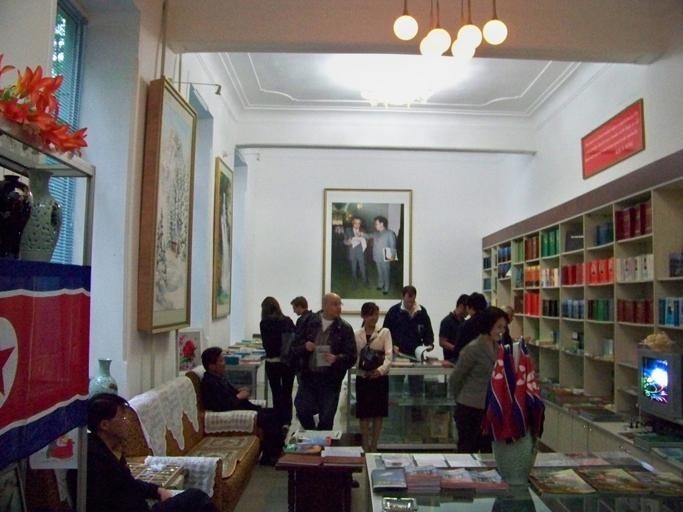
[335,356,457,450]
[0,118,97,512]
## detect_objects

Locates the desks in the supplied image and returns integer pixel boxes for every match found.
[365,451,683,512]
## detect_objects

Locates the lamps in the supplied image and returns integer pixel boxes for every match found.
[392,0,508,60]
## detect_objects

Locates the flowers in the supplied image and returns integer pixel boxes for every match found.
[0,54,90,160]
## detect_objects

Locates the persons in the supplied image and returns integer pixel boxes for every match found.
[353,303,393,452]
[291,291,358,430]
[260,297,295,415]
[343,216,369,287]
[87,394,217,511]
[383,285,434,353]
[358,214,397,294]
[440,290,513,452]
[202,346,282,463]
[290,297,315,389]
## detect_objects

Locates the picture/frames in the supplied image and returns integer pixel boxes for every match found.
[211,156,234,320]
[137,78,198,337]
[322,188,413,316]
[176,328,202,375]
[581,98,645,180]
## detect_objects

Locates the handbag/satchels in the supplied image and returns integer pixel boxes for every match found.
[280,317,297,370]
[359,328,384,370]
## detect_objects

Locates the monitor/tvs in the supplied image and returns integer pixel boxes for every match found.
[636,349,683,422]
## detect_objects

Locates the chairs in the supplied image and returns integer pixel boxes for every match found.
[185,365,267,458]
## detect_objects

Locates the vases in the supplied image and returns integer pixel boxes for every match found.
[0,174,33,259]
[20,169,62,262]
[88,358,119,396]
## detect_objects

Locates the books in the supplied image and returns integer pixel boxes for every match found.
[390,345,454,368]
[312,345,332,367]
[282,431,683,495]
[485,199,683,422]
[223,335,264,365]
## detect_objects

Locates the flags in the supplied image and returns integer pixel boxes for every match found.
[483,333,545,442]
[0,261,89,469]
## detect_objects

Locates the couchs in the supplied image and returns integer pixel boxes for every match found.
[87,375,263,512]
